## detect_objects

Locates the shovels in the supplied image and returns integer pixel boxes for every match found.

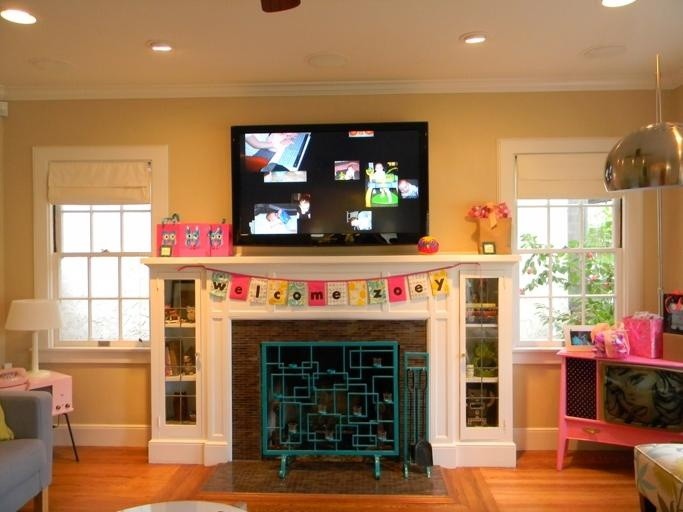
[414,368,433,467]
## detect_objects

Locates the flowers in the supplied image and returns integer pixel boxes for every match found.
[468,201,511,222]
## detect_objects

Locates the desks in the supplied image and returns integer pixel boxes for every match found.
[553,350,683,472]
[0,370,80,462]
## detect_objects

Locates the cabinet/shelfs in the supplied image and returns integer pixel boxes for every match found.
[458,269,507,441]
[155,269,203,438]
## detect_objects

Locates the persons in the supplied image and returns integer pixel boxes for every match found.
[396,179,417,197]
[606,363,682,433]
[371,162,392,204]
[345,164,354,180]
[347,211,371,231]
[244,132,298,150]
[263,209,290,231]
[294,194,310,219]
[571,330,588,346]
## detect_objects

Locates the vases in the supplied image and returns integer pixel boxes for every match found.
[478,217,510,254]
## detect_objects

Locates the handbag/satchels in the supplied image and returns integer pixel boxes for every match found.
[155,222,235,257]
[591,315,664,359]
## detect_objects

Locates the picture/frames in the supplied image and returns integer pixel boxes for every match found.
[562,323,600,353]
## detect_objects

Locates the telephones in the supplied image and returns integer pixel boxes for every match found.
[0,367,28,388]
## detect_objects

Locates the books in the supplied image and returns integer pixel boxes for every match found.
[465,299,497,323]
[164,345,173,376]
[167,340,184,376]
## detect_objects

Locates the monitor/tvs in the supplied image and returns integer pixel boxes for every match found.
[230,121,430,247]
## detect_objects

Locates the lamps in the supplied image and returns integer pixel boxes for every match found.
[604,50,683,335]
[2,298,62,378]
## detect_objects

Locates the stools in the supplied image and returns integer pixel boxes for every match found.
[631,442,683,512]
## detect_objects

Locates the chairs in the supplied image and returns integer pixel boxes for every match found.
[1,387,53,511]
